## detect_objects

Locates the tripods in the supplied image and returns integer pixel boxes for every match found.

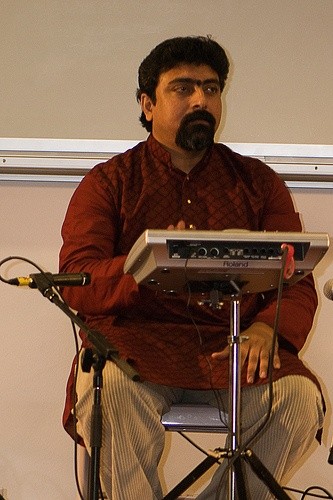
[167,282,294,500]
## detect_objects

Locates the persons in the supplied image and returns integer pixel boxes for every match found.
[58,36,327,500]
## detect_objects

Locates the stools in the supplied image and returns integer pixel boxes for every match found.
[76,404,230,499]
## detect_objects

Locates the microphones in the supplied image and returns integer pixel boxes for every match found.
[7,271,92,288]
[323,279,333,301]
